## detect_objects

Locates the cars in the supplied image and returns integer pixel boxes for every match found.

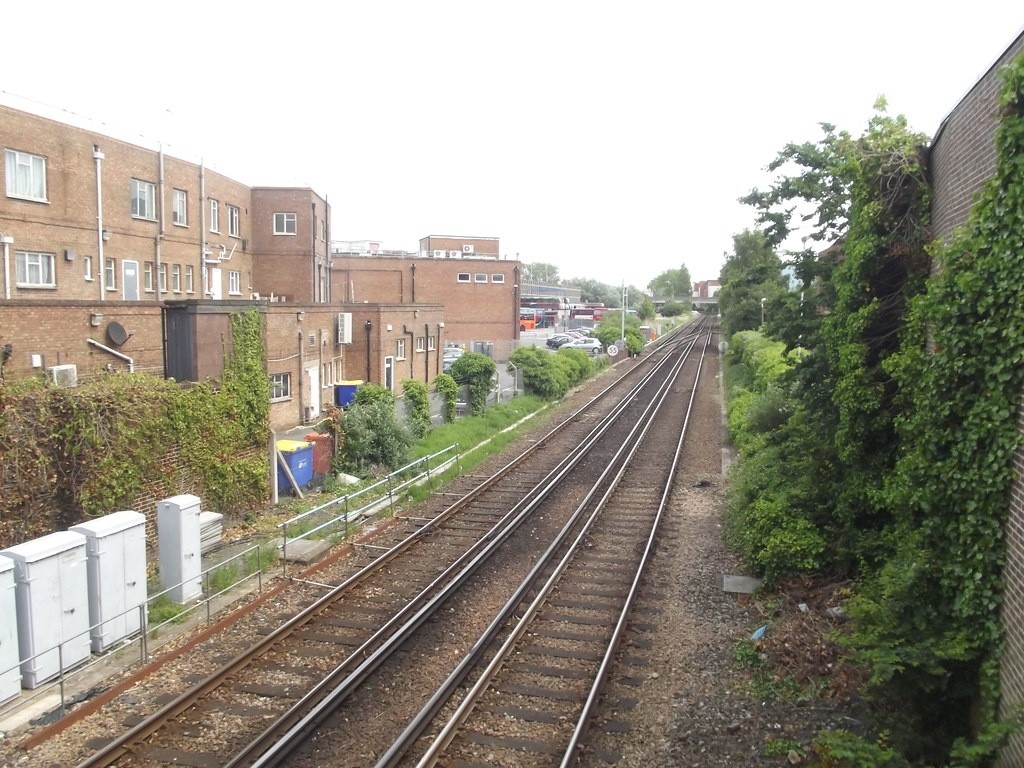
[546,327,594,350]
[559,338,602,354]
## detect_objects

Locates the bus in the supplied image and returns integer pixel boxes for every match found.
[520,295,637,332]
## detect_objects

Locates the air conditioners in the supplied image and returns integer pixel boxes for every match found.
[449,251,461,258]
[463,245,474,253]
[45,363,78,389]
[433,249,446,257]
[339,313,352,344]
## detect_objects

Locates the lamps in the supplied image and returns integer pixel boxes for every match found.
[90,314,102,327]
[103,230,114,242]
[297,312,305,320]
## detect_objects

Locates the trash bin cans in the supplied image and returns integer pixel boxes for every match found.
[335,380,364,407]
[304,432,333,474]
[275,440,316,496]
